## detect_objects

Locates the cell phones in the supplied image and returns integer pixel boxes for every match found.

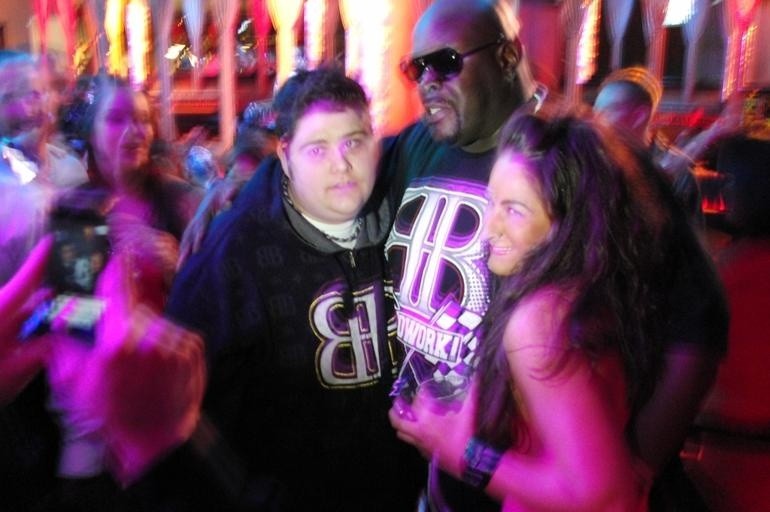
[39,190,118,347]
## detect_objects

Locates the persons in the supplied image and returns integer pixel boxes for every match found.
[0,40,769,512]
[170,0,732,512]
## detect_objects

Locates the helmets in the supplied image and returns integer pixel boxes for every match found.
[401,44,496,81]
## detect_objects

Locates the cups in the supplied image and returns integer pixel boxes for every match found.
[414,373,470,417]
